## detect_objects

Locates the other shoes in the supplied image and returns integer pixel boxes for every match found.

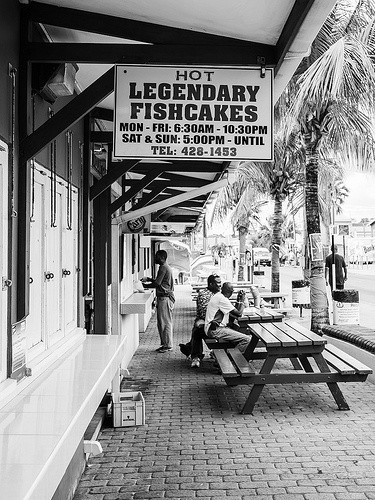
[156,346,173,352]
[213,362,220,368]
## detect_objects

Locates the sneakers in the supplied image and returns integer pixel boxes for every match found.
[190,357,200,368]
[186,354,192,362]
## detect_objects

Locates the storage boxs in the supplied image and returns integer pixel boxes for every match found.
[111,391,146,427]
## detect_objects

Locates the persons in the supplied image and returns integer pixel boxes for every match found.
[140,250,176,353]
[204,282,251,375]
[190,275,221,368]
[325,245,347,292]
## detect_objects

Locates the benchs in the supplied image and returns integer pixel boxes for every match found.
[205,338,228,350]
[212,347,255,385]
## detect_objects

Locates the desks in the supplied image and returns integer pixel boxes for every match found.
[250,291,286,310]
[237,307,285,327]
[319,343,373,382]
[243,321,349,413]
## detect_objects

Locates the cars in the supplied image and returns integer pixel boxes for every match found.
[261,256,271,266]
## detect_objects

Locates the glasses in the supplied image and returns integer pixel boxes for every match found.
[212,272,220,276]
